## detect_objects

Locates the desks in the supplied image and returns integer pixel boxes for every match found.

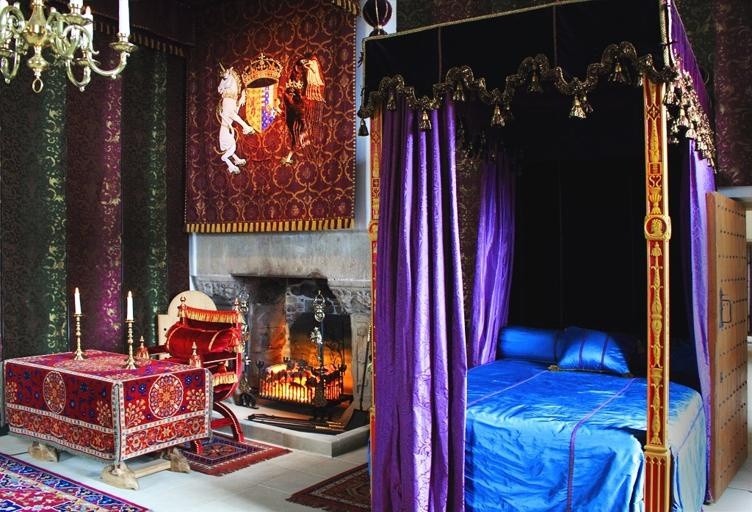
[4,347,214,487]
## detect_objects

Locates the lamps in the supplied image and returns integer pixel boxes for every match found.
[0,0,137,93]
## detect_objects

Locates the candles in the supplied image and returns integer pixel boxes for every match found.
[127,290,133,320]
[74,287,82,314]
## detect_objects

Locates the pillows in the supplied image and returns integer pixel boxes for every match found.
[498,324,691,378]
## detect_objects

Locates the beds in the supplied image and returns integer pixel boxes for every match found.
[362,0,722,512]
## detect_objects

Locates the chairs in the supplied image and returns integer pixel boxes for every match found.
[131,293,243,456]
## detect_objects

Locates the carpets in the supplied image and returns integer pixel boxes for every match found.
[286,461,372,512]
[157,430,291,476]
[0,452,154,512]
